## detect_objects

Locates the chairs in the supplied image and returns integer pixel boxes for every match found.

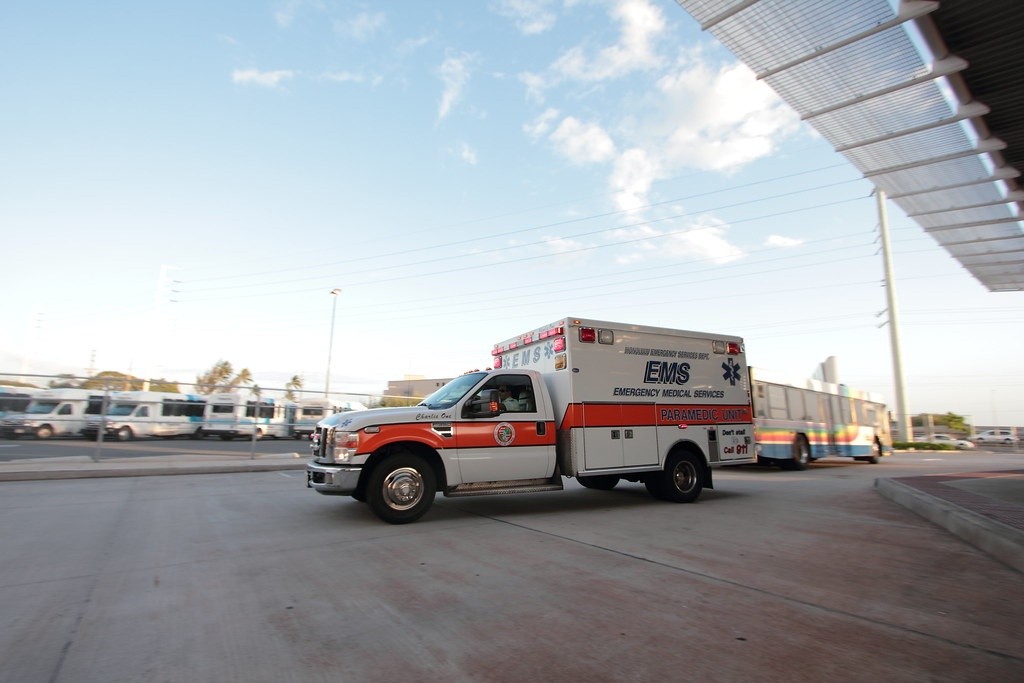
[480,389,503,412]
[518,389,534,411]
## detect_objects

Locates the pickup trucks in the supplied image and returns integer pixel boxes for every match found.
[304,318,759,522]
[0,383,367,442]
[319,282,344,422]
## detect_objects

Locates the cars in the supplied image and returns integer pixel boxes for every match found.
[914,434,975,452]
[968,429,1019,446]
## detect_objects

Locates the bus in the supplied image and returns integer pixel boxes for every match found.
[749,364,894,469]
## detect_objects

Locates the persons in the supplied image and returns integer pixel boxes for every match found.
[489,383,520,411]
